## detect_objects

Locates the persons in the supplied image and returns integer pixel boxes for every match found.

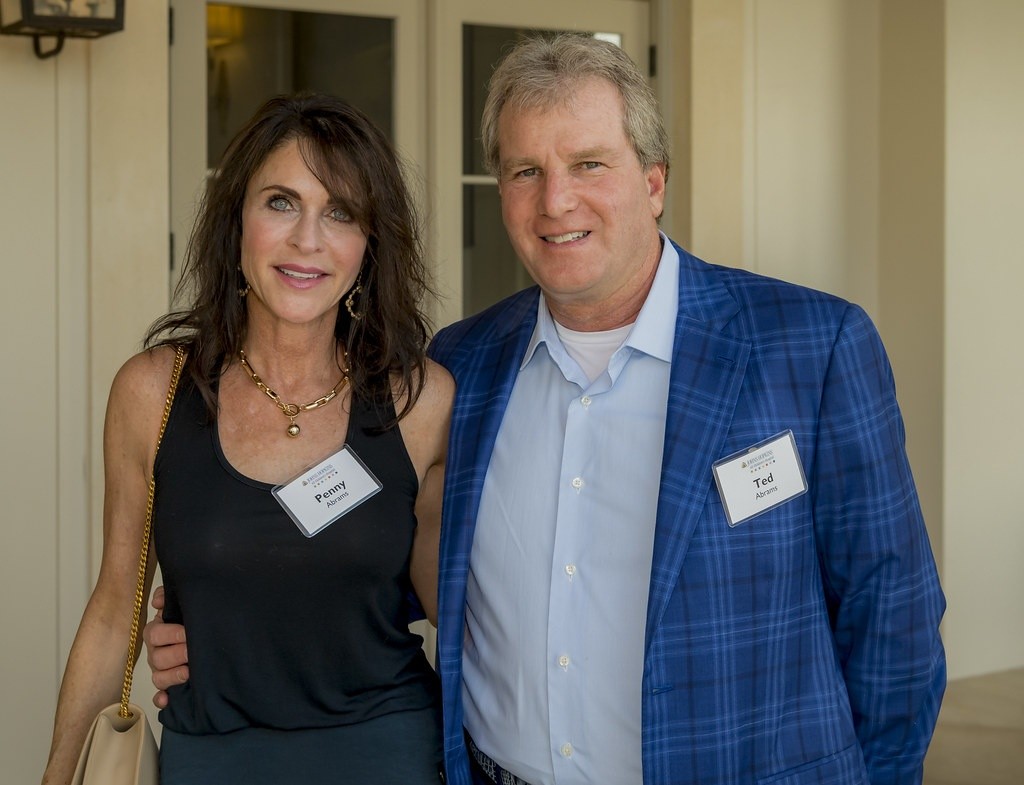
[144,34,947,784]
[39,90,457,784]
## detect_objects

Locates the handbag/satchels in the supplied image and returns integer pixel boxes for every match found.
[70,701,160,785]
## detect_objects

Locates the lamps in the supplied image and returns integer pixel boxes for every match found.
[0,0,125,59]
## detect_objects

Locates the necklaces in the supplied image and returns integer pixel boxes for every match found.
[237,342,352,437]
